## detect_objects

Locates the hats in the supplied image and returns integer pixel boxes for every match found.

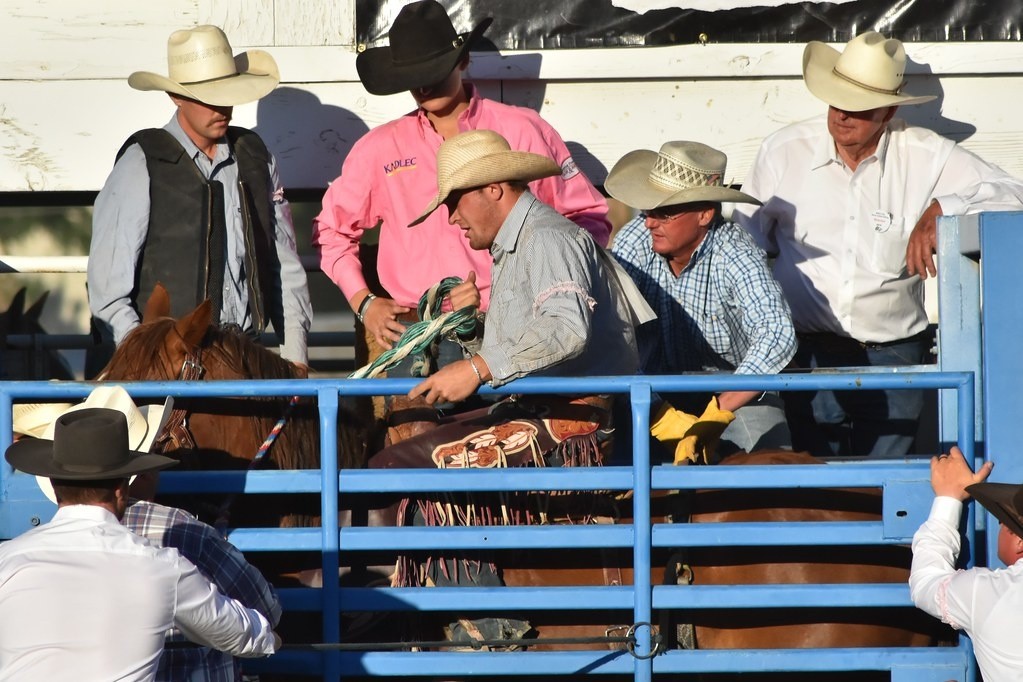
[964,482,1023,540]
[802,31,937,111]
[35,374,174,506]
[407,129,563,228]
[129,25,279,105]
[604,141,765,210]
[4,409,180,479]
[356,0,493,95]
[12,380,73,440]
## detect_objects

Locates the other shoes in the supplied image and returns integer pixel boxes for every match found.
[442,617,529,653]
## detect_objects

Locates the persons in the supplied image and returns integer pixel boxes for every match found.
[310,0,613,420]
[341,130,648,643]
[731,30,1023,459]
[55,401,283,682]
[610,140,797,465]
[909,445,1023,682]
[0,409,283,682]
[84,25,314,383]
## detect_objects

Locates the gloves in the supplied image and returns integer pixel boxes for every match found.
[649,401,699,456]
[674,396,736,465]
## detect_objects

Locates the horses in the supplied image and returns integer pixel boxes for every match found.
[96,282,942,652]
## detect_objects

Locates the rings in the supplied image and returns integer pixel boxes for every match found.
[938,456,947,461]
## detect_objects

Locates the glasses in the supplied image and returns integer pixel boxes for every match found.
[641,209,690,224]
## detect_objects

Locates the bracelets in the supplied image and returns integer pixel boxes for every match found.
[356,293,378,325]
[468,357,485,386]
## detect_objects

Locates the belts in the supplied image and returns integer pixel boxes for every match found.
[796,332,925,352]
